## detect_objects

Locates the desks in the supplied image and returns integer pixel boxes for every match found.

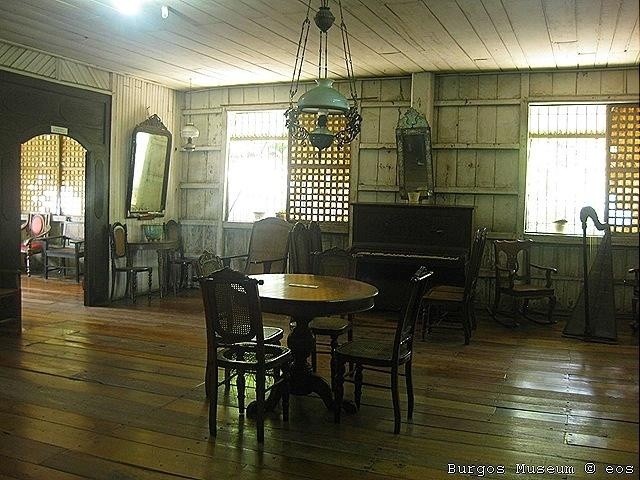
[225,273,380,420]
[122,238,179,299]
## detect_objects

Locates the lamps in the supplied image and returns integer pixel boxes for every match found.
[284,0,363,149]
[180,122,198,150]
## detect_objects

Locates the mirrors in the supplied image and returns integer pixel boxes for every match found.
[125,113,172,218]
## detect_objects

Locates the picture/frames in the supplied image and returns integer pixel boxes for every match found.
[394,106,434,203]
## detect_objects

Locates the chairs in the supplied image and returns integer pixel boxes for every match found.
[217,217,290,276]
[420,229,480,346]
[305,221,321,264]
[162,220,200,291]
[628,268,640,332]
[35,221,85,284]
[290,223,345,319]
[21,212,52,277]
[333,267,435,433]
[485,237,558,329]
[202,266,291,444]
[288,245,355,377]
[195,250,283,389]
[430,229,485,331]
[109,221,153,305]
